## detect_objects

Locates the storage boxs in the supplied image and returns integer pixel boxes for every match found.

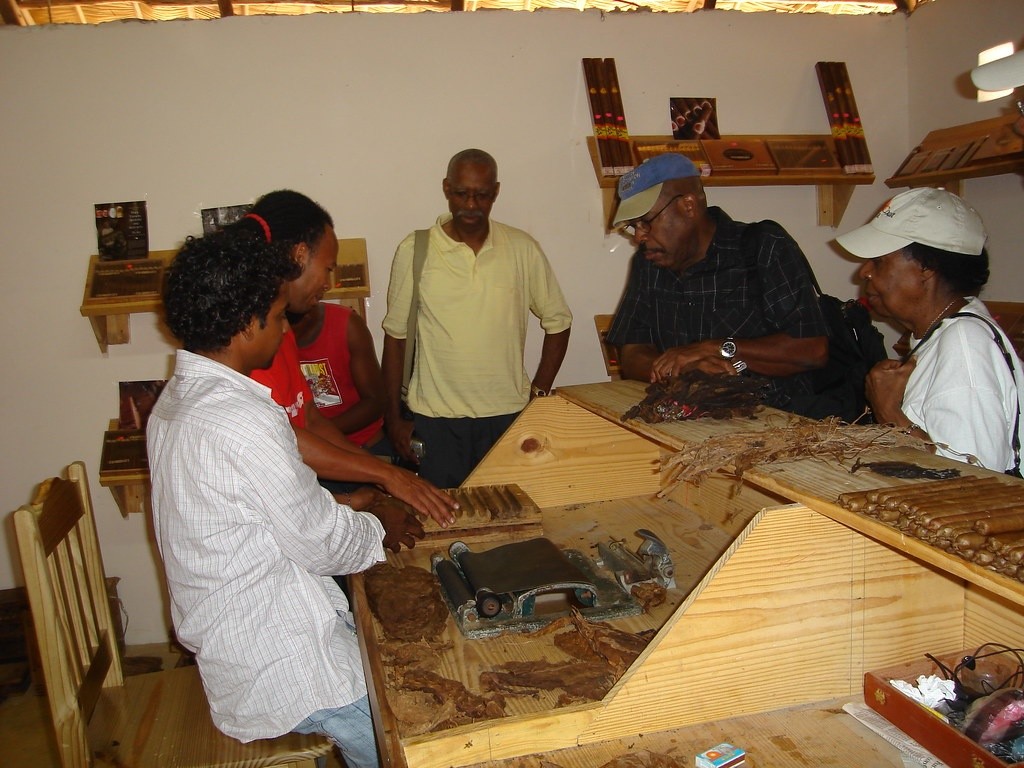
[862,646,1024,768]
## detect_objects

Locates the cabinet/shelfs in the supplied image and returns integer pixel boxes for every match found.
[80,238,370,516]
[883,109,1024,357]
[586,128,877,379]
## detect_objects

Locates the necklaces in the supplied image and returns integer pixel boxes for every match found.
[922,296,958,338]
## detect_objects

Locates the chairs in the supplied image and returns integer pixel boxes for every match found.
[13,460,334,768]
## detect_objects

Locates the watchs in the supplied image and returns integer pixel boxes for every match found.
[720,336,748,375]
[530,382,546,397]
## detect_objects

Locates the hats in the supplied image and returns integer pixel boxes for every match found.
[836,187,987,258]
[612,153,700,228]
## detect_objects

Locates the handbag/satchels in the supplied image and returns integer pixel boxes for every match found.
[740,219,890,427]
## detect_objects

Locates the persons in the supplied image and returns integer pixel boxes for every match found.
[221,187,461,529]
[833,186,1024,479]
[381,148,574,492]
[602,152,839,422]
[143,226,425,768]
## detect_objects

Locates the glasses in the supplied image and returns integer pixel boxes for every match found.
[623,194,684,235]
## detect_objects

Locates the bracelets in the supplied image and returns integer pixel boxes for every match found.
[345,491,351,507]
[899,421,919,436]
